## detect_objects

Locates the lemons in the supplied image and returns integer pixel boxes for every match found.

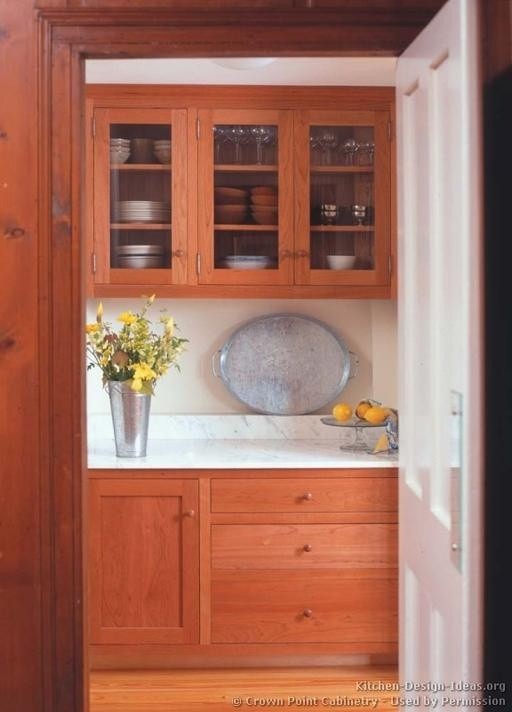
[357,403,372,420]
[363,407,391,424]
[332,402,353,422]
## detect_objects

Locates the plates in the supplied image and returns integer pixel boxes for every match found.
[211,313,359,415]
[110,200,171,223]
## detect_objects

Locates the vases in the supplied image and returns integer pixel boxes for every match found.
[106,378,156,458]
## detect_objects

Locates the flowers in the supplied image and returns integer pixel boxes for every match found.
[84,294,192,397]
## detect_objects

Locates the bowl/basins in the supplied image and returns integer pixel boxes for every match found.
[326,255,357,271]
[110,136,171,163]
[113,245,168,269]
[316,201,371,226]
[214,186,278,225]
[224,254,269,270]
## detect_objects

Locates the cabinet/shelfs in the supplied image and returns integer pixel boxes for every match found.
[192,83,395,299]
[82,470,202,673]
[202,470,400,668]
[84,83,191,300]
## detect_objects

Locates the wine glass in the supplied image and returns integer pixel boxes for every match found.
[212,126,277,166]
[310,128,375,166]
